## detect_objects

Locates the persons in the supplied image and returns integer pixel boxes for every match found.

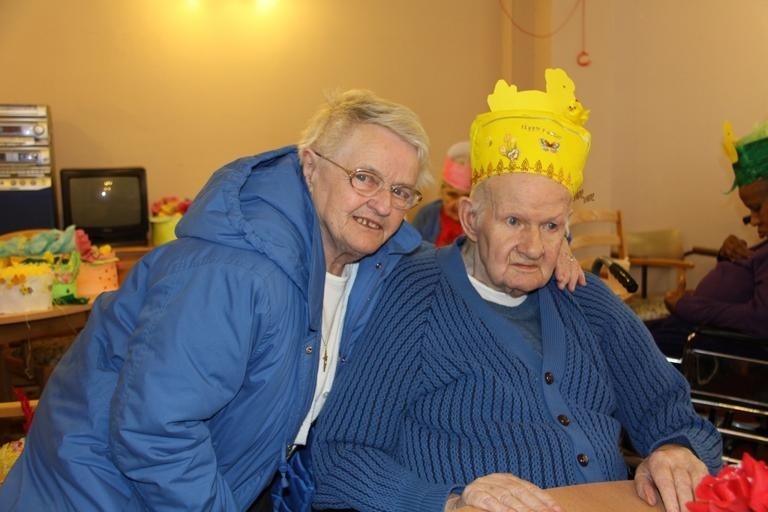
[310,64,723,512]
[643,122,768,358]
[0,87,587,511]
[411,140,472,248]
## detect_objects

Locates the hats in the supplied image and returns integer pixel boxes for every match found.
[469,67,591,199]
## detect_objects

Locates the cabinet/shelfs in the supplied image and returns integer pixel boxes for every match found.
[0,311,92,401]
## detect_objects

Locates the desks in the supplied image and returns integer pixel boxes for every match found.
[451,481,666,512]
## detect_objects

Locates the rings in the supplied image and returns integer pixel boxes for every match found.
[570,259,577,262]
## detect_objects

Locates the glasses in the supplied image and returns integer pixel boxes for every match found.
[312,147,424,212]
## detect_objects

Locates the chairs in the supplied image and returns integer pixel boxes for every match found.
[565,209,693,321]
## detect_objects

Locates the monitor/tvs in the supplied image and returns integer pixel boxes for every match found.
[60,168,149,247]
